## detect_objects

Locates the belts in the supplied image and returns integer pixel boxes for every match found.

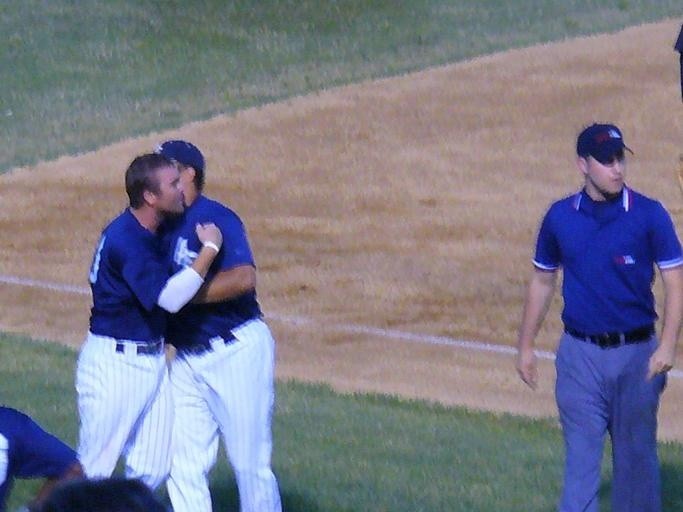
[171,324,239,361]
[562,319,656,349]
[114,340,166,357]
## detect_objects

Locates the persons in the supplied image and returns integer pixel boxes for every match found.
[70,152,222,497]
[0,405,85,512]
[154,138,285,512]
[513,119,683,509]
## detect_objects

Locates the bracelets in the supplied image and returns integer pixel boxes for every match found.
[203,242,221,254]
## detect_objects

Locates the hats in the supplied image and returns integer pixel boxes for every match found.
[155,138,206,170]
[575,119,634,164]
